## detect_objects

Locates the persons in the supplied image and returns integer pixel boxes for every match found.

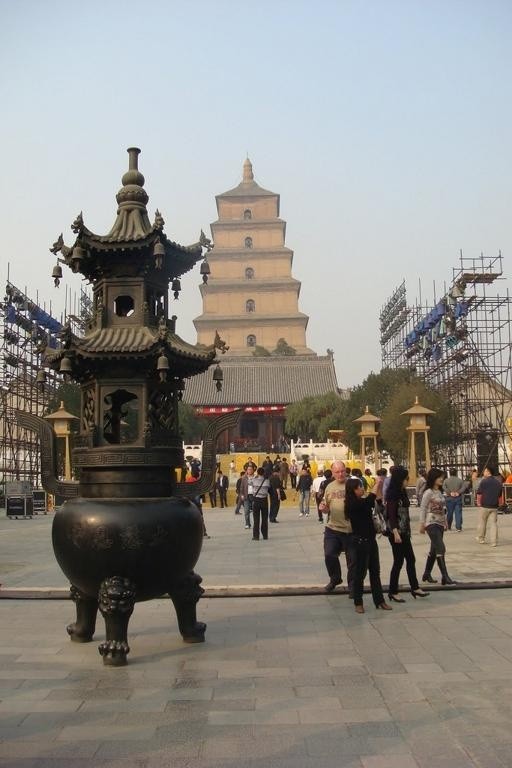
[419,468,456,585]
[382,469,430,602]
[178,454,478,541]
[476,466,502,547]
[344,476,393,613]
[319,461,358,599]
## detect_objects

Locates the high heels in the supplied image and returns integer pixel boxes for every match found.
[356,604,364,613]
[375,602,392,609]
[411,590,429,599]
[388,593,405,602]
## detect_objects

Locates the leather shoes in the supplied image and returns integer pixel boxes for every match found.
[326,579,343,590]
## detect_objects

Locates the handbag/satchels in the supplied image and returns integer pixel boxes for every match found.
[280,490,286,500]
[382,499,408,534]
[373,500,386,533]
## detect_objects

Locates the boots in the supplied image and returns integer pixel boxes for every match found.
[422,556,437,583]
[435,556,456,585]
